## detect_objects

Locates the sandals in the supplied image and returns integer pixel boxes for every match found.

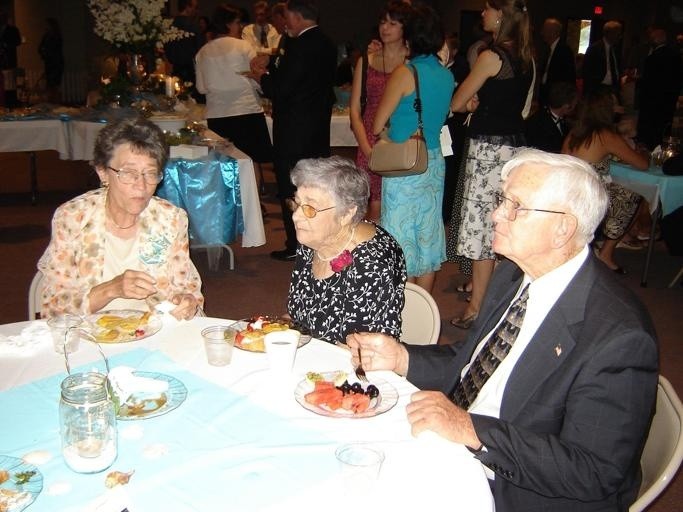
[453,284,474,304]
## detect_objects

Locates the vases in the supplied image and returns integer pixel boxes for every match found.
[130,51,146,96]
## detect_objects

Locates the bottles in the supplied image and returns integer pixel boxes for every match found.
[59,371,119,474]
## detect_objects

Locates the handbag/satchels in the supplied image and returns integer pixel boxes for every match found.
[349,54,369,132]
[366,62,429,179]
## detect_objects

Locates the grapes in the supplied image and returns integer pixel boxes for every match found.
[335,381,379,398]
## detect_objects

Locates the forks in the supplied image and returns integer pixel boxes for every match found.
[353,327,370,383]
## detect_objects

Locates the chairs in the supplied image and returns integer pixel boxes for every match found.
[626,373,683,512]
[397,280,443,346]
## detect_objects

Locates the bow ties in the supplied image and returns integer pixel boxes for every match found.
[548,109,563,126]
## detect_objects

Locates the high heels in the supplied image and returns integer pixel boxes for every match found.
[450,311,479,329]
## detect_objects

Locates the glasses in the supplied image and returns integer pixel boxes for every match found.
[104,165,166,186]
[492,191,568,222]
[283,196,338,220]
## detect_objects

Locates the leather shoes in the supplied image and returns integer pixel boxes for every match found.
[613,232,662,251]
[268,246,299,263]
[611,266,633,278]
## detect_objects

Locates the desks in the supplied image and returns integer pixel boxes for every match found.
[0,94,358,271]
[602,154,682,288]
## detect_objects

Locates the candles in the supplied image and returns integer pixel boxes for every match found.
[165,76,177,98]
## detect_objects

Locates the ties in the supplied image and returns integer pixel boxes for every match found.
[608,46,619,88]
[448,282,533,412]
[260,26,269,50]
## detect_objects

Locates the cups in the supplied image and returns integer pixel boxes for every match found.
[265,330,301,374]
[201,326,239,367]
[47,312,83,353]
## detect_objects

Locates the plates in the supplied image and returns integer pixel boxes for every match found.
[1,451,43,512]
[294,370,400,419]
[114,369,189,420]
[0,105,209,122]
[224,314,313,353]
[82,309,163,342]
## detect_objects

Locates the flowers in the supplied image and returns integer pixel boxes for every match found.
[82,2,197,53]
